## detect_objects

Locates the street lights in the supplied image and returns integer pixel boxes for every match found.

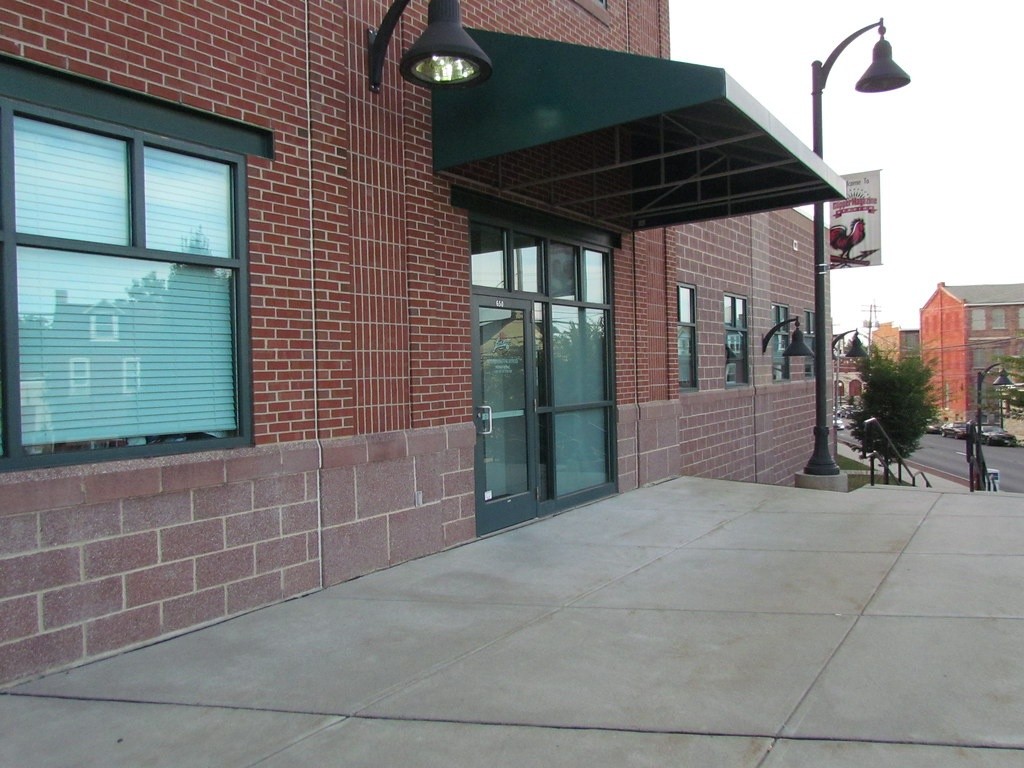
[792,16,911,494]
[832,329,869,457]
[975,362,1014,489]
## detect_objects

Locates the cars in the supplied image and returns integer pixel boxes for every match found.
[836,405,853,419]
[923,419,967,439]
[833,420,845,430]
[976,423,1016,446]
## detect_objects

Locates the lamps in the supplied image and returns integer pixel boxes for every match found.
[762,315,814,357]
[832,328,868,360]
[366,0,492,93]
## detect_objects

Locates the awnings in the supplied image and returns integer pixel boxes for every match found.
[432,26,847,234]
